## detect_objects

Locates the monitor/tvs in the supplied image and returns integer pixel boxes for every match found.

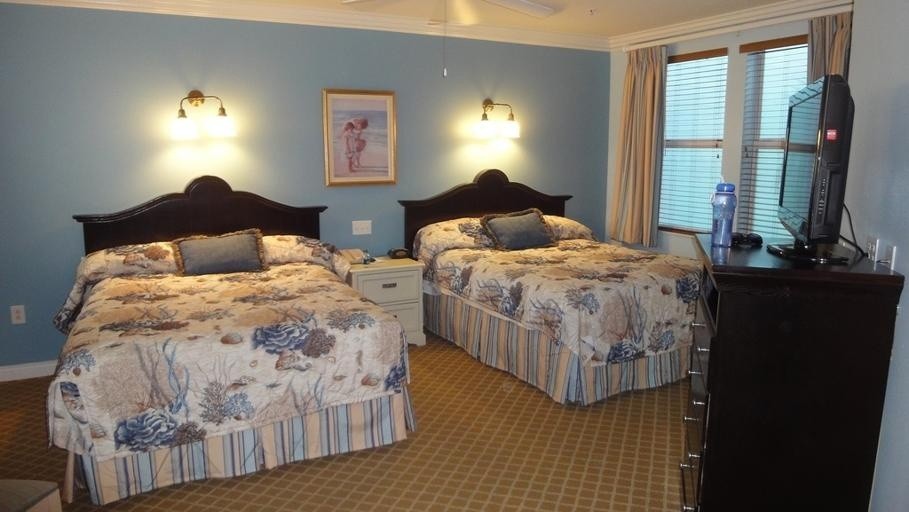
[767,75,855,265]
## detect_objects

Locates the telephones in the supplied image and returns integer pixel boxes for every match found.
[339,247,365,265]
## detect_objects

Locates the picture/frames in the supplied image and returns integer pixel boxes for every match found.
[321,88,398,184]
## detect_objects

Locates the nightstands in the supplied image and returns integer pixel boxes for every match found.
[339,254,427,351]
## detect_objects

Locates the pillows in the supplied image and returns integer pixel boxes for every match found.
[173,226,272,275]
[482,207,561,250]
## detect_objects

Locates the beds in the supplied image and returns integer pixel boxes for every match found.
[45,174,416,507]
[397,167,707,408]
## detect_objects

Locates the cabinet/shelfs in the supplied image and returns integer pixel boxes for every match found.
[678,226,908,508]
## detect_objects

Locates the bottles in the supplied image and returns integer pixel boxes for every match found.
[362,250,371,265]
[711,183,737,248]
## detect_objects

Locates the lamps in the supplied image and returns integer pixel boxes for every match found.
[456,98,525,167]
[164,88,241,172]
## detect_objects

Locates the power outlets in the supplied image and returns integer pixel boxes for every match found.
[863,233,878,267]
[885,241,897,277]
